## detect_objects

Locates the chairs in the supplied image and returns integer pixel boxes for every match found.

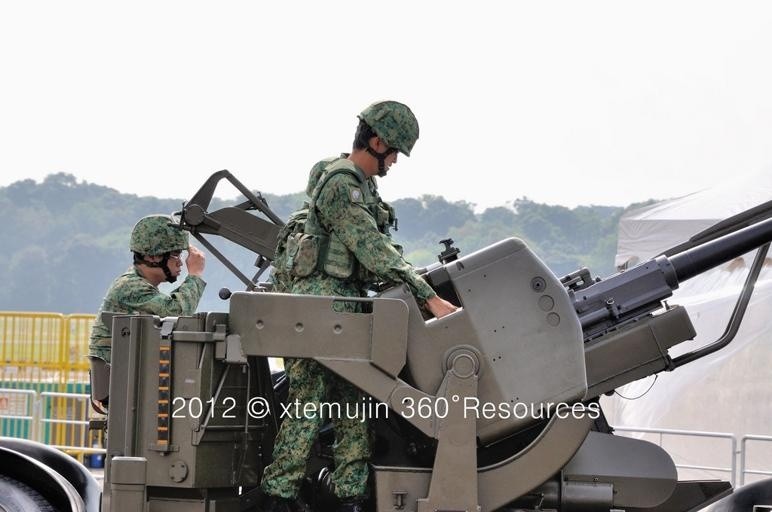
[86,355,108,434]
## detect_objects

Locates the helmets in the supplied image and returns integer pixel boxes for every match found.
[129,214,190,257]
[356,101,418,157]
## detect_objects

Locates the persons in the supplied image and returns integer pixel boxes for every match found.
[86,213,208,412]
[258,98,459,512]
